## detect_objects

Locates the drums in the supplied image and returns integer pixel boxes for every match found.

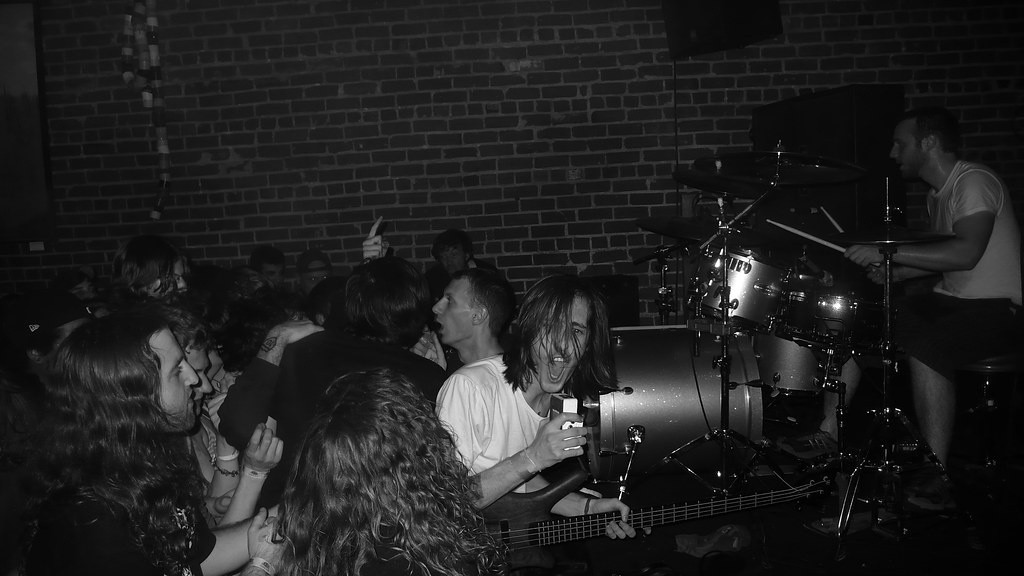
[751,333,823,397]
[685,247,786,335]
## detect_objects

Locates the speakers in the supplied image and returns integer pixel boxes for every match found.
[661,0,783,59]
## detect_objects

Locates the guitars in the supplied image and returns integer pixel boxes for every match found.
[481,470,832,574]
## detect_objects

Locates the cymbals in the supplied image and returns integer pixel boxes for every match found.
[693,150,869,186]
[824,229,960,246]
[631,216,772,249]
[673,167,760,198]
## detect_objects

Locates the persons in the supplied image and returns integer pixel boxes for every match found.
[0,231,518,528]
[0,308,288,576]
[433,274,645,540]
[270,372,511,576]
[779,105,1024,513]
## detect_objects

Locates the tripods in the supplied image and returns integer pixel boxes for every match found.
[642,177,976,576]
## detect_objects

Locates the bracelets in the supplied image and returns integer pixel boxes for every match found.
[248,556,277,575]
[510,451,536,481]
[240,465,271,479]
[217,450,240,461]
